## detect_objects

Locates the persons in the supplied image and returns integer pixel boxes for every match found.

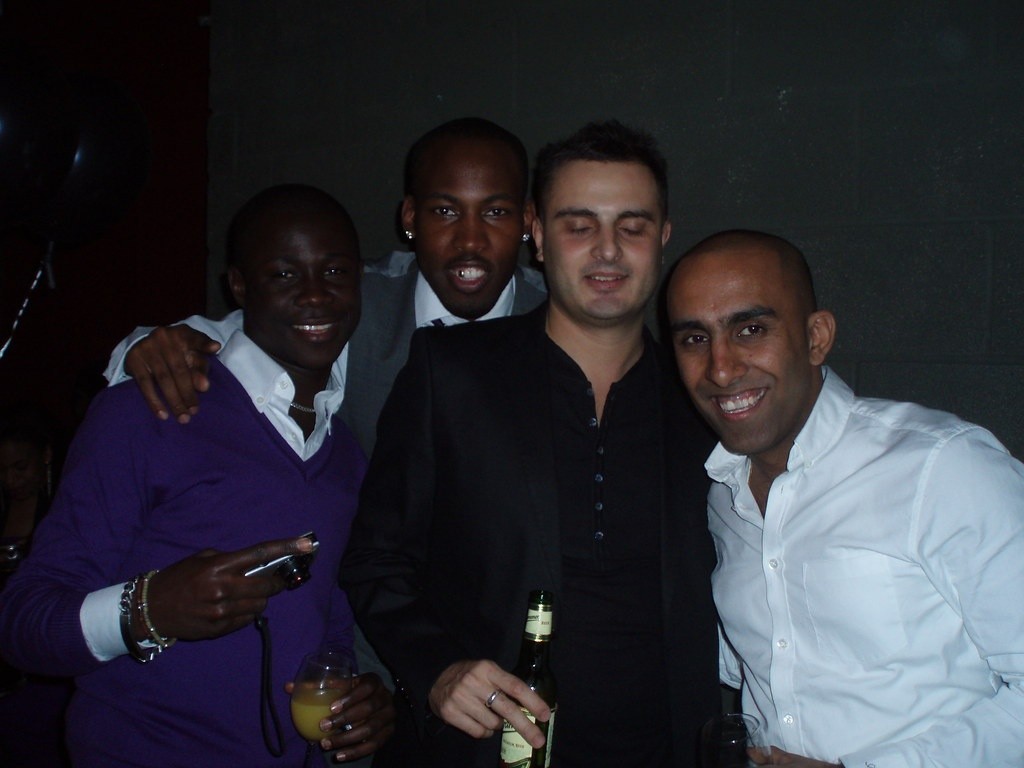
[101,117,548,768]
[656,228,1023,768]
[341,119,721,768]
[0,183,398,768]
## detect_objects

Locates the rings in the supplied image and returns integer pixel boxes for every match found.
[484,688,502,709]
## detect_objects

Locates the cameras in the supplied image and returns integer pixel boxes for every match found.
[244,531,321,591]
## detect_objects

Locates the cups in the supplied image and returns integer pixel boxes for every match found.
[698,713,771,768]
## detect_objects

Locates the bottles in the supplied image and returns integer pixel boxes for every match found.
[499,590,559,768]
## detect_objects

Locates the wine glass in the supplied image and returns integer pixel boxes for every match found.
[284,652,351,768]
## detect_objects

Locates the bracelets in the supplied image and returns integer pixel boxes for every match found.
[120,568,179,666]
